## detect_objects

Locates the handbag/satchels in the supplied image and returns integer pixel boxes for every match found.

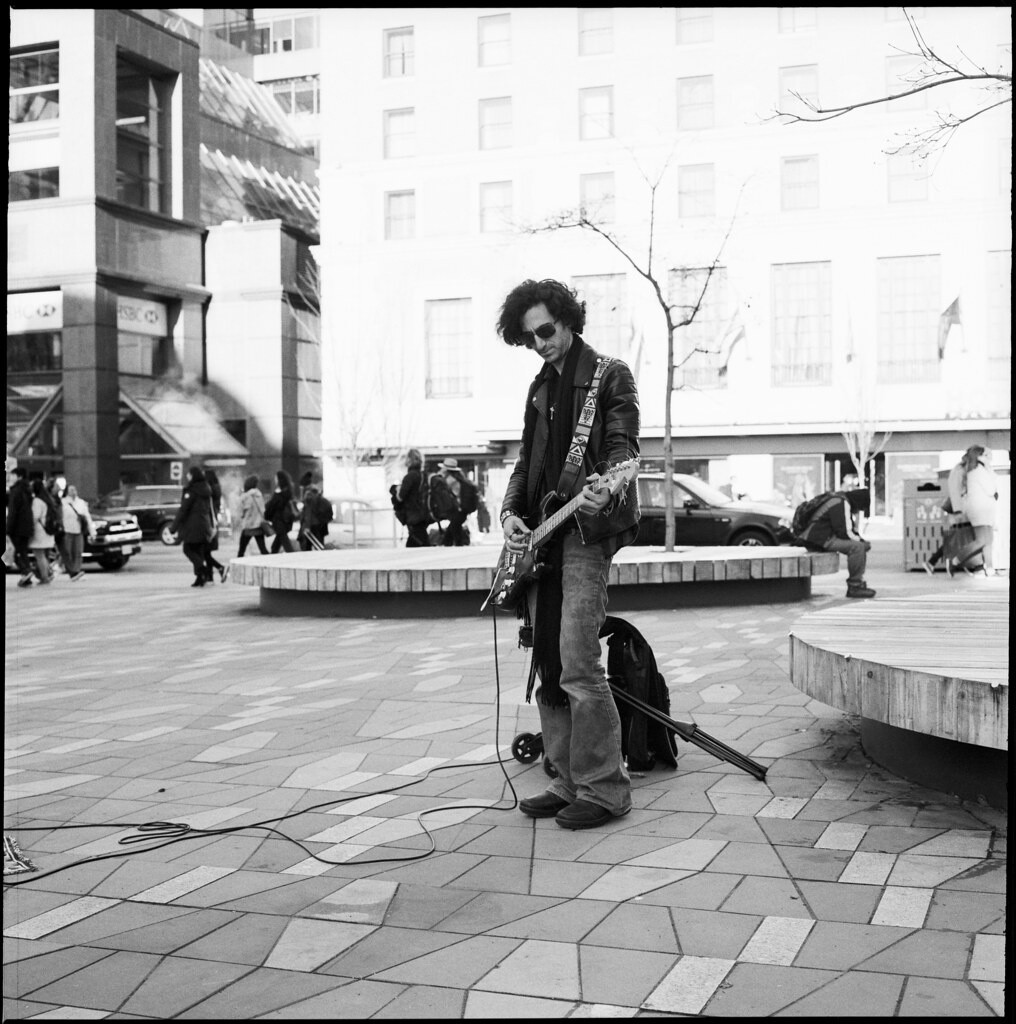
[77,513,90,539]
[945,512,994,569]
[262,521,274,536]
[207,518,220,543]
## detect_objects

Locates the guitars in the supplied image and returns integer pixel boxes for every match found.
[490,454,642,611]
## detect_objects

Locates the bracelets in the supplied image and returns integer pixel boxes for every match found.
[499,509,516,526]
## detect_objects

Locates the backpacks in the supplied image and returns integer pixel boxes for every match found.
[283,498,302,523]
[793,490,850,536]
[304,486,334,525]
[595,613,678,770]
[38,496,64,536]
[419,469,457,524]
[451,474,481,515]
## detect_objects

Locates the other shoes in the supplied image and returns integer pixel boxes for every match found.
[848,585,876,598]
[68,571,84,581]
[922,561,934,577]
[20,575,35,587]
[519,790,570,817]
[220,565,230,582]
[555,799,613,828]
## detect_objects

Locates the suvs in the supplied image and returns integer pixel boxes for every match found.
[6,489,142,571]
[84,485,231,545]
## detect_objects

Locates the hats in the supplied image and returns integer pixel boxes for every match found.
[438,458,462,471]
[243,474,260,492]
[299,471,312,487]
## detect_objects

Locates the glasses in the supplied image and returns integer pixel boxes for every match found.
[521,316,562,348]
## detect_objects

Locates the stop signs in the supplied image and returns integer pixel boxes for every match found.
[169,462,185,480]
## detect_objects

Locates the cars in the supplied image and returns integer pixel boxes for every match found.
[634,474,806,548]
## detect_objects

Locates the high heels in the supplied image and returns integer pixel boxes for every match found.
[193,576,206,587]
[197,566,211,577]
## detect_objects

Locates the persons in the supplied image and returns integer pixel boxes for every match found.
[236,475,269,557]
[170,466,214,587]
[47,474,71,574]
[293,471,324,551]
[922,445,982,576]
[943,445,1003,581]
[198,469,229,583]
[435,458,475,546]
[265,470,295,553]
[6,468,36,587]
[57,485,98,581]
[393,448,434,547]
[795,486,876,601]
[30,479,58,585]
[495,280,645,834]
